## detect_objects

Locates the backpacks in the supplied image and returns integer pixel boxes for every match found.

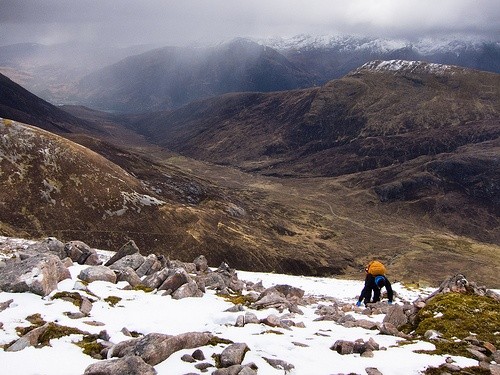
[367,261,386,275]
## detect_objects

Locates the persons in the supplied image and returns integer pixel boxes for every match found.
[356,273,394,308]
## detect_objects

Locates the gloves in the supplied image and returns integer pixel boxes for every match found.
[356,301,361,306]
[388,302,392,304]
